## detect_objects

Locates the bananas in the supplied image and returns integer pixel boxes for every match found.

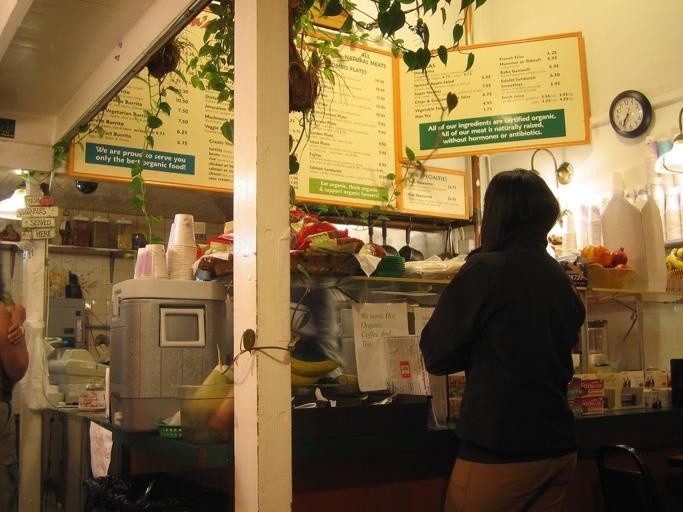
[336,375,358,385]
[665,246,683,271]
[291,356,338,387]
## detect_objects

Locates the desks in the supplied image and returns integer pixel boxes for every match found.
[668,455,683,467]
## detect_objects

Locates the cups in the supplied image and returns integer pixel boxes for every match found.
[561,129,683,254]
[131,212,196,279]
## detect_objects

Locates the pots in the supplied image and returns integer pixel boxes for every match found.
[360,214,459,261]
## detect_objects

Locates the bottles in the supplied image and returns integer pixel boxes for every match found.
[73,213,132,250]
[182,364,234,440]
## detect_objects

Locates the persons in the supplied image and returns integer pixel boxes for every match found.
[0,262,29,512]
[421,167,587,512]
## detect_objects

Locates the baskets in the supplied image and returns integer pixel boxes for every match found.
[202,254,233,276]
[297,249,359,275]
[155,421,183,438]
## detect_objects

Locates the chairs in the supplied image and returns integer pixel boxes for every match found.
[595,444,662,512]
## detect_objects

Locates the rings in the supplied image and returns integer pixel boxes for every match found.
[20,326,25,336]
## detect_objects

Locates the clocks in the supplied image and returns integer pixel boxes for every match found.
[608,91,652,138]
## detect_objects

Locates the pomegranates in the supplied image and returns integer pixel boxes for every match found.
[582,246,632,269]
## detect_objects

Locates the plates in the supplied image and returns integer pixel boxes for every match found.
[356,256,405,277]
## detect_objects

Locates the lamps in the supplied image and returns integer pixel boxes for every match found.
[531,149,574,189]
[654,108,683,175]
[0,181,29,220]
